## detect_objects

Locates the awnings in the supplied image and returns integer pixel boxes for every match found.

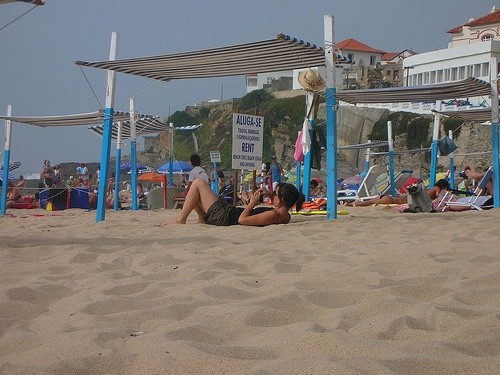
[302,76,493,104]
[87,115,202,144]
[431,107,493,124]
[73,31,339,83]
[0,109,155,128]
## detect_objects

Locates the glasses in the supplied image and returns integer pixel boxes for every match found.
[272,191,280,198]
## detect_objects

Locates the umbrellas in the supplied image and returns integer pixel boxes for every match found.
[116,161,148,172]
[157,161,194,175]
[136,171,164,183]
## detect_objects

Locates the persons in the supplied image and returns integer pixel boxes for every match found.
[183,154,209,195]
[16,175,25,190]
[25,159,145,210]
[299,179,318,200]
[354,179,450,209]
[4,182,23,209]
[210,164,225,197]
[266,155,285,192]
[156,178,305,226]
[444,165,492,196]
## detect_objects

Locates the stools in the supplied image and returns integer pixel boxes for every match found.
[173,198,185,209]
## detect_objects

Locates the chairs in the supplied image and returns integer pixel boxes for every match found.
[436,166,493,212]
[360,170,413,199]
[311,165,380,202]
